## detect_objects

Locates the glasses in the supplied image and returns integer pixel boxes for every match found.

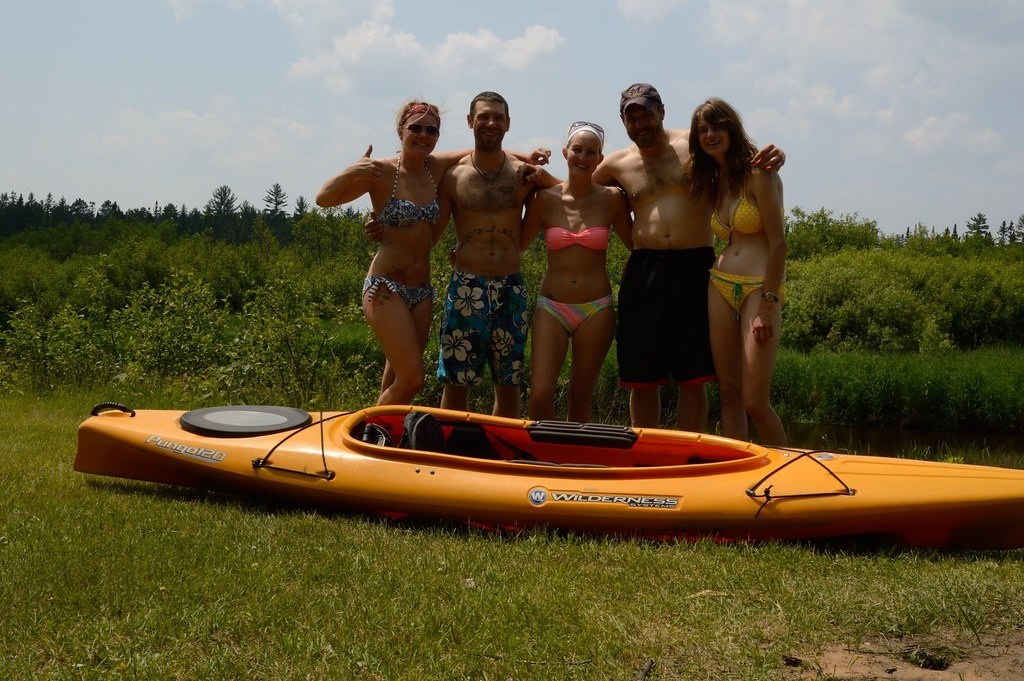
[568,120,604,146]
[402,124,439,135]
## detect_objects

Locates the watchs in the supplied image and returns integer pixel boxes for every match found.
[762,292,779,303]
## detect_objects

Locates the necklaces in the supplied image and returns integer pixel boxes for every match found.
[471,150,506,184]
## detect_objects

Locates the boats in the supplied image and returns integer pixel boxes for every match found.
[70,405,1024,552]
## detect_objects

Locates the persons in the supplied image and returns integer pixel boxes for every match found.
[447,125,634,423]
[514,84,786,433]
[316,100,551,406]
[684,98,789,447]
[364,92,562,419]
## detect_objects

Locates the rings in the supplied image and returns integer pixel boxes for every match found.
[369,233,372,236]
[377,172,380,176]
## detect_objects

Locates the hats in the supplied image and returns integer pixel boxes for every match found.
[567,123,604,153]
[619,83,662,115]
